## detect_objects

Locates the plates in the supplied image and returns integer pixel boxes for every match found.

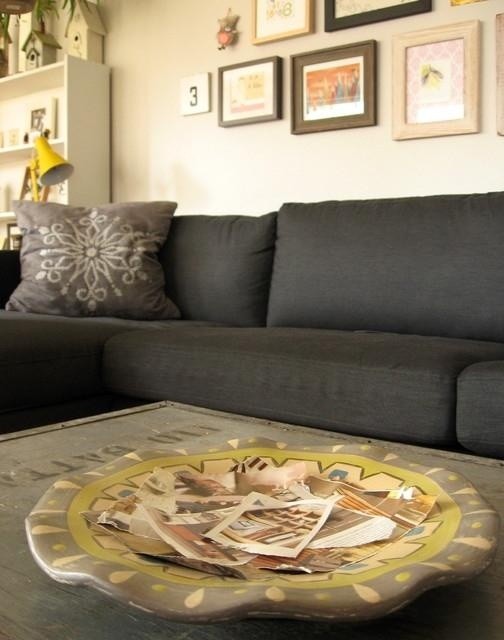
[25,435,501,627]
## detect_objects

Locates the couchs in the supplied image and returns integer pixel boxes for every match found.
[0,190,503,457]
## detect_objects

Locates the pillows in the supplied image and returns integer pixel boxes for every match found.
[4,199,178,319]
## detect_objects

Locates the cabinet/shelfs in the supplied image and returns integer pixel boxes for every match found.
[0,55,111,250]
